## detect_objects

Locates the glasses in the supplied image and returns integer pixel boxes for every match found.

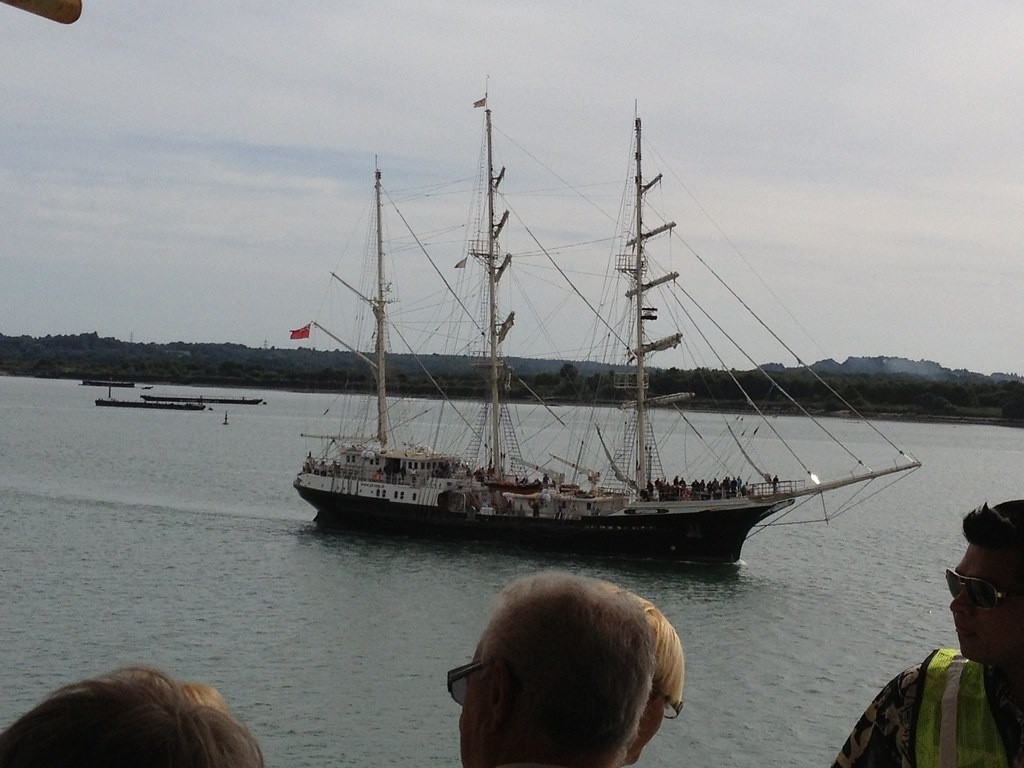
[648,684,680,718]
[446,657,494,705]
[945,571,1015,609]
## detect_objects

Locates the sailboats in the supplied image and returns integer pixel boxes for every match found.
[293,110,923,563]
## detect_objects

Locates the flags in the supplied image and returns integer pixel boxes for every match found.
[289,324,311,340]
[474,98,485,107]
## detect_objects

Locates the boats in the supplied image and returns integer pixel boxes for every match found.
[94,398,206,410]
[140,395,263,405]
[83,380,134,387]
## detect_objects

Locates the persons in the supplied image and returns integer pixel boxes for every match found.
[400,465,405,480]
[0,666,264,768]
[830,499,1024,768]
[543,473,549,484]
[448,572,685,768]
[532,500,539,518]
[305,452,337,477]
[773,474,779,494]
[640,476,752,500]
[506,500,512,514]
[409,467,417,486]
